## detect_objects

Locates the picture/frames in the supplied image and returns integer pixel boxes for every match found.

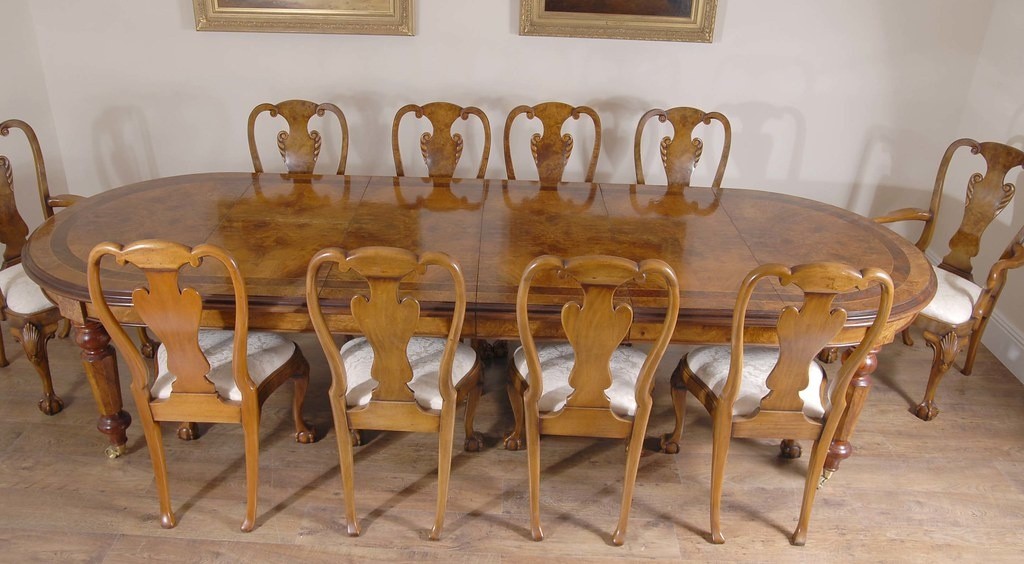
[192,0,415,36]
[518,0,719,43]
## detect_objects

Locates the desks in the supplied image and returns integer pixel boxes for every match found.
[21,171,937,489]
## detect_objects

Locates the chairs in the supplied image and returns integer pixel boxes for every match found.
[634,107,732,187]
[821,139,1023,421]
[246,98,348,175]
[306,247,487,542]
[392,102,495,360]
[494,103,601,360]
[503,254,681,547]
[0,119,162,415]
[87,241,318,532]
[660,261,894,547]
